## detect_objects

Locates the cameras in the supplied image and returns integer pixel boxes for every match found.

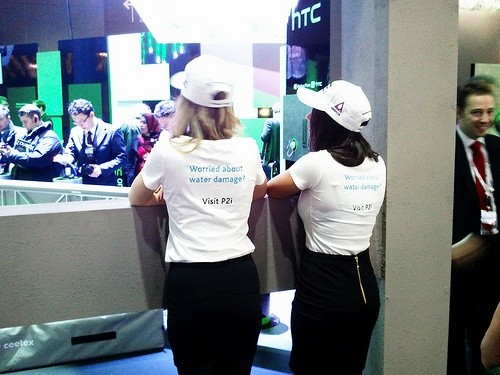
[80,163,93,174]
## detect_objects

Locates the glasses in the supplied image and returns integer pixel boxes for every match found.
[73,115,89,126]
[18,111,34,116]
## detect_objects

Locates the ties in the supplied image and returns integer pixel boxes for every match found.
[469,141,495,234]
[87,131,92,144]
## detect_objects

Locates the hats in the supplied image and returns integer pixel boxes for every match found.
[170,56,236,108]
[297,80,371,132]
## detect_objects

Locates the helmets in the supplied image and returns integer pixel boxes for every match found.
[153,100,176,118]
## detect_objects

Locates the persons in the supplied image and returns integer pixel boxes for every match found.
[267,78,387,375]
[0,96,9,108]
[129,56,266,375]
[134,112,160,177]
[154,100,177,130]
[53,98,128,187]
[0,103,27,181]
[260,102,280,175]
[449,73,499,375]
[31,99,54,130]
[1,104,66,181]
